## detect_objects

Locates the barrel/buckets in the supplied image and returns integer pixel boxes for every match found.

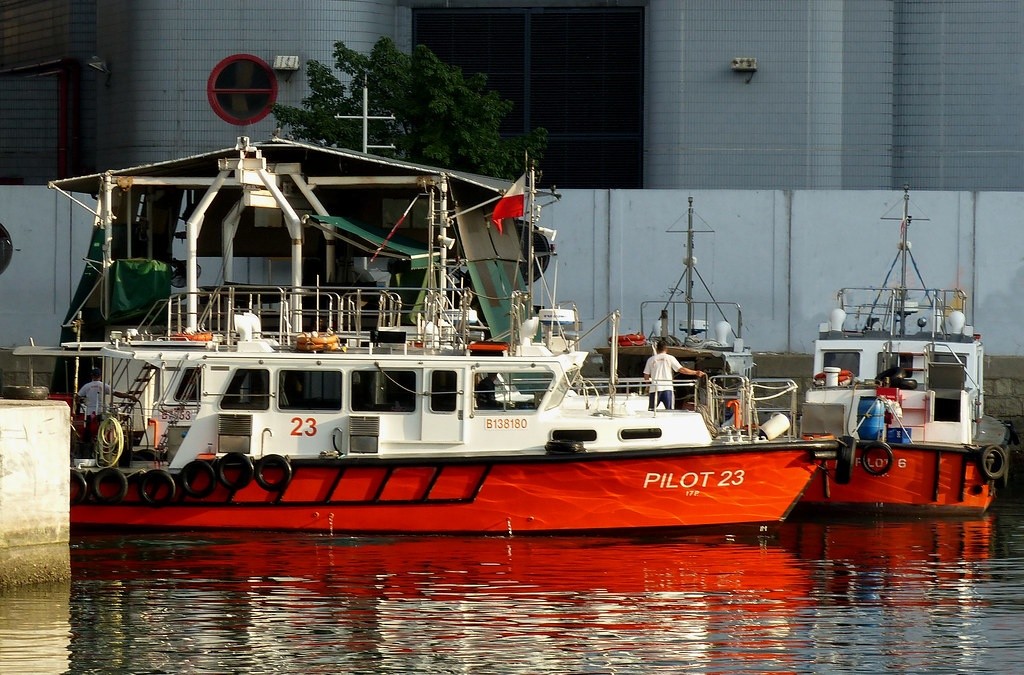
[760,413,790,441]
[857,396,884,440]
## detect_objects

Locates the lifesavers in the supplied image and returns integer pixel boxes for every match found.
[861,441,895,476]
[898,379,917,389]
[89,467,129,504]
[976,443,1009,480]
[254,454,293,492]
[176,460,216,500]
[607,333,646,346]
[170,332,212,341]
[834,435,857,485]
[814,369,852,384]
[297,333,340,351]
[874,365,907,388]
[215,451,254,491]
[136,469,176,508]
[544,439,585,455]
[2,385,52,400]
[70,467,89,508]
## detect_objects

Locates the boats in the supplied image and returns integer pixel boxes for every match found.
[793,182,1012,516]
[597,196,756,407]
[818,517,1000,595]
[67,537,817,672]
[15,132,843,536]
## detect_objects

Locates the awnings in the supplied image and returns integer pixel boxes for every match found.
[310,215,441,269]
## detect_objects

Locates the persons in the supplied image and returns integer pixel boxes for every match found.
[643,340,705,410]
[75,369,138,440]
[476,372,504,410]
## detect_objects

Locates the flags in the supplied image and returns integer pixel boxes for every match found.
[491,173,525,237]
